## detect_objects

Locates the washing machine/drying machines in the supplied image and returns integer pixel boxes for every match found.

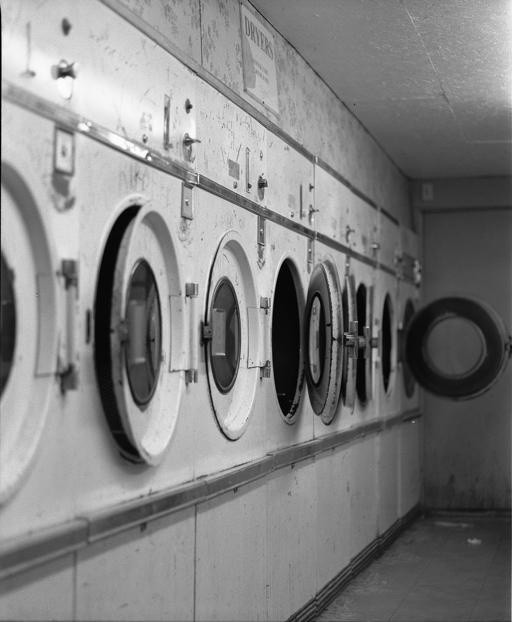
[2,1,510,622]
